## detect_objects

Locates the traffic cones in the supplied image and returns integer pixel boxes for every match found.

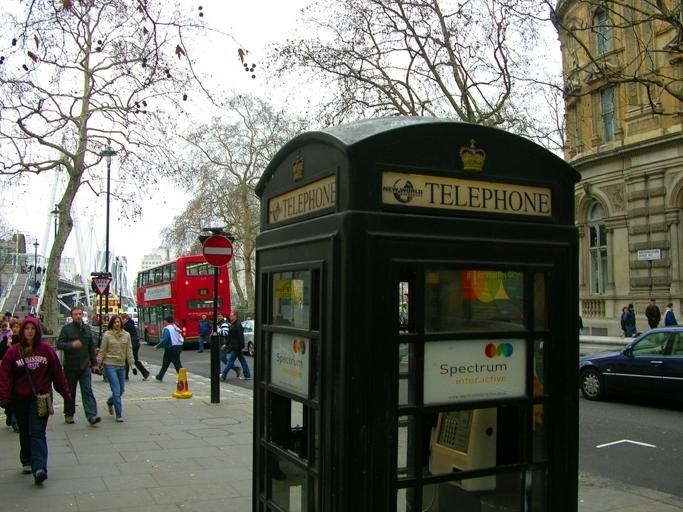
[172,366,192,399]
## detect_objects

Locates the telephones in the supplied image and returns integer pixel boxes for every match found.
[421,403,496,493]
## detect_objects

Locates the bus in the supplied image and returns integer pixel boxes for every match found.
[95,291,122,331]
[136,254,230,346]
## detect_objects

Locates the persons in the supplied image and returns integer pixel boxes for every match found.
[19,263,46,315]
[0,312,72,487]
[620,298,678,336]
[56,306,150,425]
[197,310,251,380]
[152,315,183,383]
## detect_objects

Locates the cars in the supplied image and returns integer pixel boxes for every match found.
[240,319,256,356]
[124,312,138,328]
[579,325,682,400]
[59,311,90,327]
[398,301,408,324]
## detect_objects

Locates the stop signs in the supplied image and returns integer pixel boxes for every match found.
[202,234,232,267]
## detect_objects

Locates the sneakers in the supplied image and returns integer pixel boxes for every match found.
[65,414,76,425]
[219,367,252,381]
[89,416,102,425]
[143,373,162,383]
[107,404,116,416]
[116,416,124,422]
[22,466,32,474]
[33,469,48,488]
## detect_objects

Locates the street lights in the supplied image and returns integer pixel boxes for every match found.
[50,204,59,327]
[31,238,39,315]
[119,260,123,299]
[98,137,116,332]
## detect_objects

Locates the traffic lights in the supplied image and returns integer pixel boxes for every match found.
[117,299,120,308]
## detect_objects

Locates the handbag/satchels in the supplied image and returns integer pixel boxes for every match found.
[37,393,54,417]
